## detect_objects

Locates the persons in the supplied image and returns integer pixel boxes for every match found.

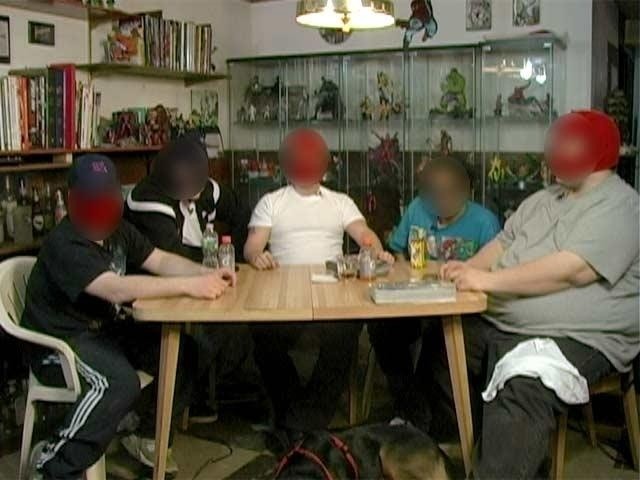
[18,155,236,480]
[433,110,640,480]
[123,138,248,426]
[242,127,395,454]
[366,156,501,432]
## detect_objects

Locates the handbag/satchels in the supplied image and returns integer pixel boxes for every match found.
[270,406,463,480]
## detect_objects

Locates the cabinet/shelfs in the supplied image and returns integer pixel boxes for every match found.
[227,39,567,258]
[0,3,231,256]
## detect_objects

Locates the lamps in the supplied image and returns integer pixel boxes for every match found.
[295,0,395,32]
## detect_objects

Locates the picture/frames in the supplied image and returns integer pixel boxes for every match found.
[0,16,10,63]
[513,1,540,26]
[465,1,492,31]
[29,20,54,46]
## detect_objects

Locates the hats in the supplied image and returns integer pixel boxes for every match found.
[68,154,117,191]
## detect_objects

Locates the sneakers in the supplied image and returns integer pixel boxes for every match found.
[122,434,178,475]
[190,407,217,424]
[24,441,50,480]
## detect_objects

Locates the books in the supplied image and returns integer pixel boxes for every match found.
[119,15,212,74]
[0,62,102,150]
[368,278,457,305]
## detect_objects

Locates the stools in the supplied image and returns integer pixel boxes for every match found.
[551,370,639,479]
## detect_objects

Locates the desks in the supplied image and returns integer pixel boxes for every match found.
[134,263,487,480]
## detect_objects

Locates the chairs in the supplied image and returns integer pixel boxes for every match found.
[1,256,154,480]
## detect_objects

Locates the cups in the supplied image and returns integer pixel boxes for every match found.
[336,255,360,283]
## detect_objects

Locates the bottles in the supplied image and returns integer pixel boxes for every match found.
[54,187,67,224]
[201,223,218,269]
[2,176,17,240]
[44,185,54,231]
[18,179,28,207]
[219,236,235,274]
[32,186,44,237]
[360,237,377,282]
